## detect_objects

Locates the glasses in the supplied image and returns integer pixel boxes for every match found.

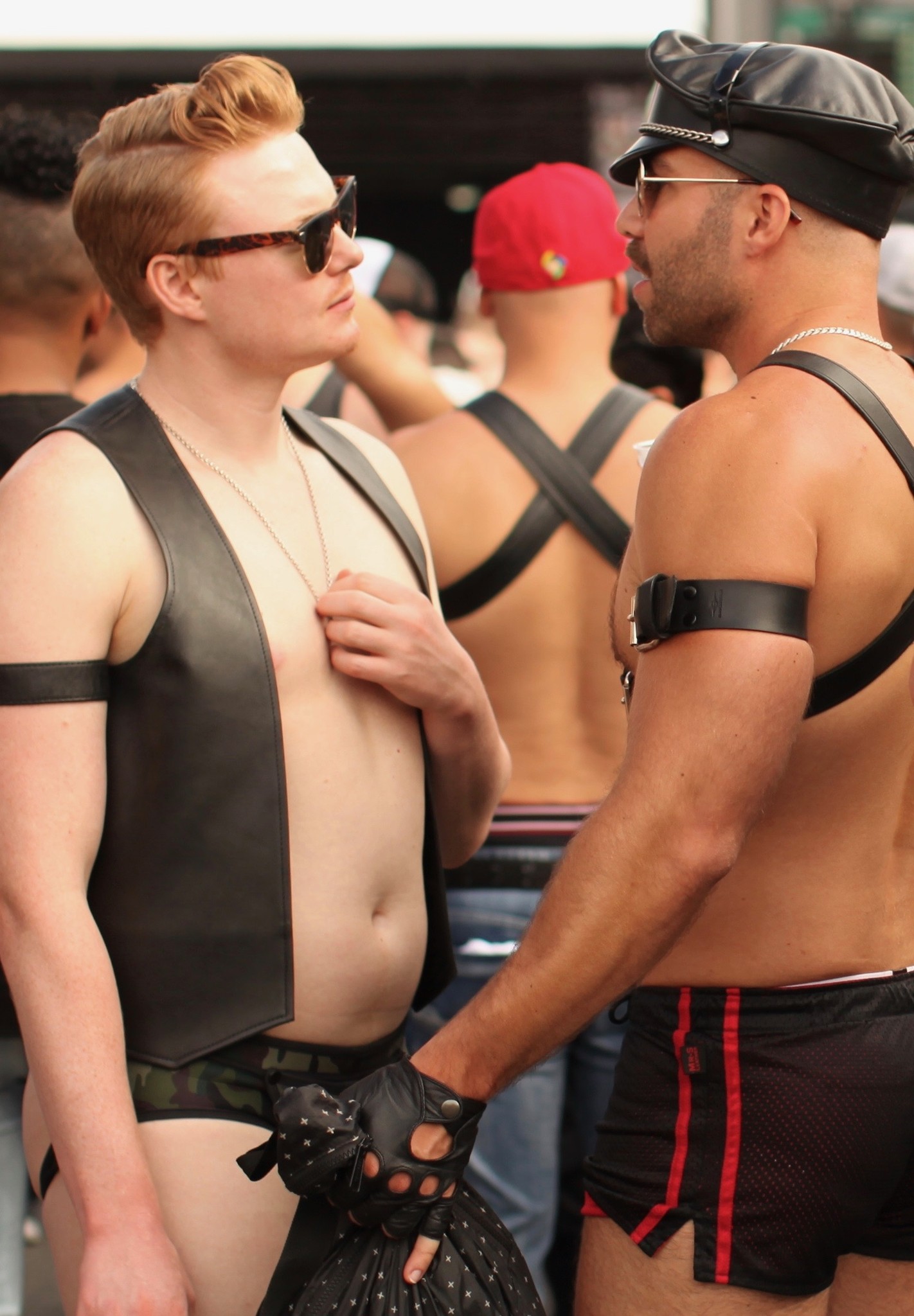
[143,176,358,275]
[633,157,805,222]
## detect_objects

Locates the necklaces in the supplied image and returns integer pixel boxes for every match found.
[131,375,332,622]
[770,327,893,356]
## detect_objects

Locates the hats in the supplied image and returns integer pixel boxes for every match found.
[607,28,914,238]
[473,163,632,291]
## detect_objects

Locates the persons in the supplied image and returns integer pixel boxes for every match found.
[0,30,914,1316]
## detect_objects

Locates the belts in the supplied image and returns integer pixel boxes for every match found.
[440,855,553,888]
